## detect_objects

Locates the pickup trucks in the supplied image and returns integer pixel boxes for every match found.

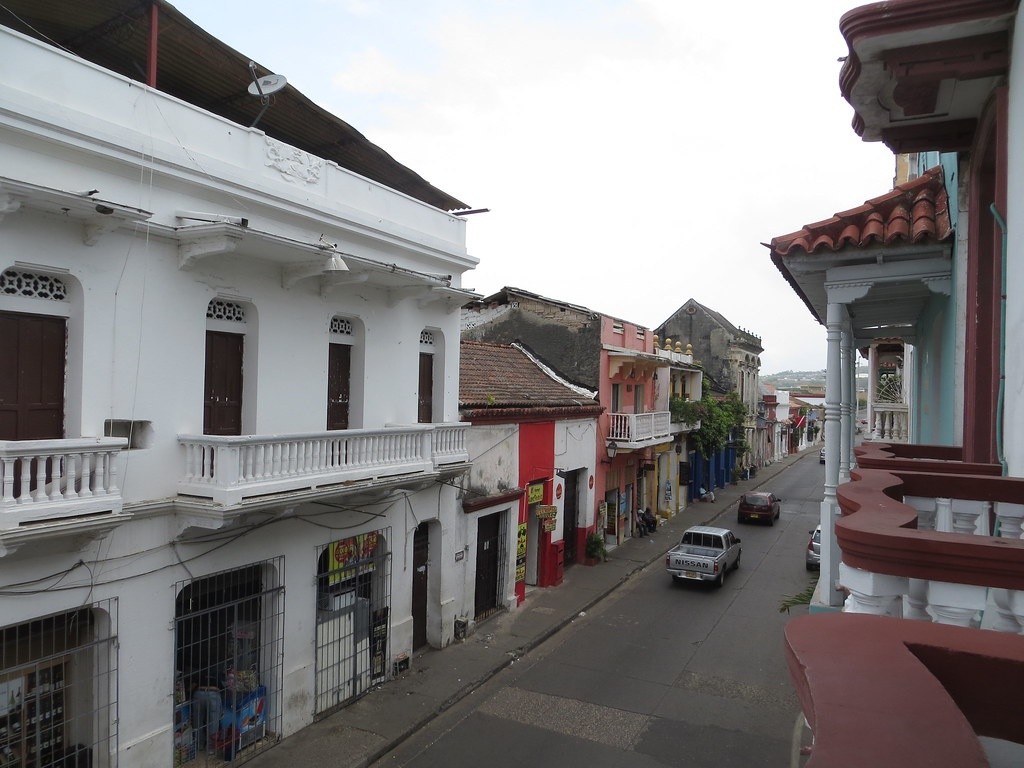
[664,526,742,588]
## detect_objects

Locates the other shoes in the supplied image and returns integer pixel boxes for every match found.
[711,500,715,503]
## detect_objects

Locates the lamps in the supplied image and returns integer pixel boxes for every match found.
[631,372,635,378]
[321,239,350,272]
[681,376,685,382]
[601,440,618,465]
[897,359,904,367]
[674,377,677,382]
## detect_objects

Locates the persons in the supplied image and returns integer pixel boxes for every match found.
[700,484,715,503]
[644,507,658,532]
[636,510,649,537]
[189,672,220,755]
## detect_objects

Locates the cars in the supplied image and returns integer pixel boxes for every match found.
[807,524,824,570]
[739,492,782,525]
[820,447,826,464]
[862,419,868,425]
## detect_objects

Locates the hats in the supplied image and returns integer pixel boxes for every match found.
[638,510,644,513]
